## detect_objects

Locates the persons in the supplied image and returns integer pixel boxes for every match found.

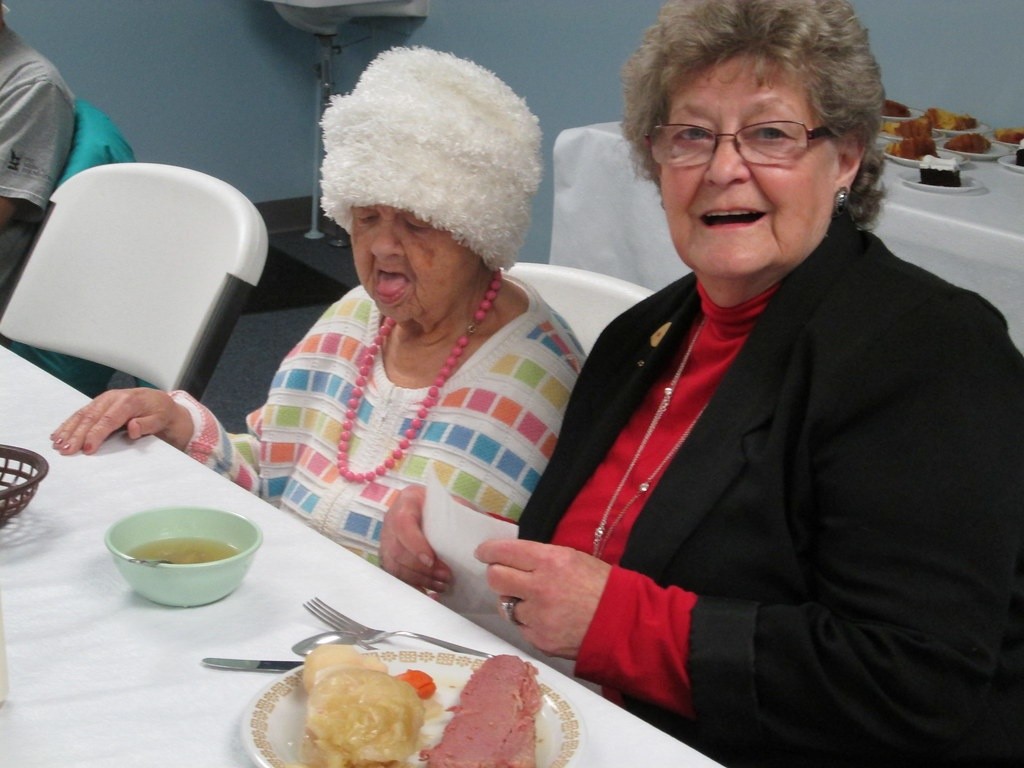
[50,48,587,698]
[473,0,1024,768]
[0,0,76,349]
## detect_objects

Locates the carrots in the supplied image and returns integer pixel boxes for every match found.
[395,670,435,699]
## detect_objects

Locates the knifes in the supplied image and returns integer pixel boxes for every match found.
[203,657,304,670]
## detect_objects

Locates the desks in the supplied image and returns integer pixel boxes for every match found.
[250,194,361,308]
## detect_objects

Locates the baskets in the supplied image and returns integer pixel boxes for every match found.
[0,444,49,523]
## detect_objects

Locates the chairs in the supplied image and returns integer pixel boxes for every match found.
[2,104,662,401]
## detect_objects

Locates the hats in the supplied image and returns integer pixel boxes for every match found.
[318,44,543,270]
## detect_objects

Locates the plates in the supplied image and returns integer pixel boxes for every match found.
[241,649,582,767]
[882,107,1024,190]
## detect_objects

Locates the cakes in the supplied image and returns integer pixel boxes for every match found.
[878,98,1024,187]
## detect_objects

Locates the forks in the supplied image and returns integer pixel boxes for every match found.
[303,597,492,657]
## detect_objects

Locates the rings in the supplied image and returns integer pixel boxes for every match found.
[501,597,523,626]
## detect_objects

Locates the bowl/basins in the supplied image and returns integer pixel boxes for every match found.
[104,506,263,608]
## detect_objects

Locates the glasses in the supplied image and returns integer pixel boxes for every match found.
[645,118,832,168]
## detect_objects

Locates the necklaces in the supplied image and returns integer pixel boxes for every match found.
[336,267,500,484]
[594,315,709,557]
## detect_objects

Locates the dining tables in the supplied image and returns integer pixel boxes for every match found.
[547,117,1023,354]
[0,335,726,768]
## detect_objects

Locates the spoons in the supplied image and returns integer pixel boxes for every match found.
[293,632,378,655]
